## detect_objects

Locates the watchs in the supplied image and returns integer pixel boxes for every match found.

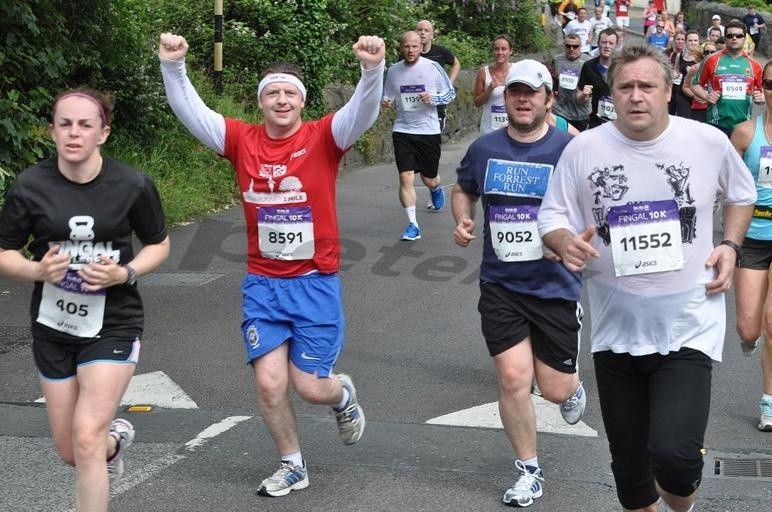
[124,264,136,287]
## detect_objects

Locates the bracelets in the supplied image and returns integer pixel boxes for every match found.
[721,240,744,263]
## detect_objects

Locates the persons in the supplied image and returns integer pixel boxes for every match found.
[535,41,757,511]
[475,0,765,232]
[409,20,463,209]
[453,60,586,506]
[729,62,772,434]
[0,85,173,512]
[380,31,456,240]
[158,32,386,498]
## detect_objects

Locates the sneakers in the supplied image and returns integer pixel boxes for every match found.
[430,187,445,210]
[256,458,308,498]
[333,374,365,445]
[756,398,772,431]
[560,386,585,426]
[503,464,543,505]
[740,336,759,357]
[402,224,418,240]
[107,419,135,486]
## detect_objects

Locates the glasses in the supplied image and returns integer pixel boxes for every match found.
[726,33,744,38]
[565,44,579,49]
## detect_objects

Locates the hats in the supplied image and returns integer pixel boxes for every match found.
[506,60,554,95]
